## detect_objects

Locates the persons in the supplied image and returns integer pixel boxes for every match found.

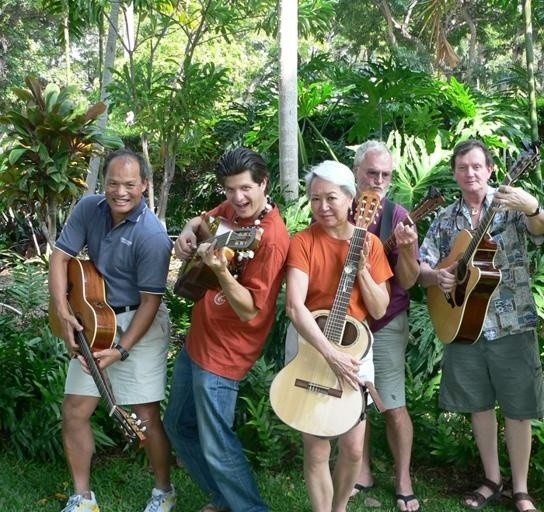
[311,139,422,512]
[283,159,395,512]
[48,151,178,512]
[160,146,290,512]
[417,140,544,511]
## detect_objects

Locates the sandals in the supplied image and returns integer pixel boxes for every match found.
[461,477,504,511]
[511,490,542,512]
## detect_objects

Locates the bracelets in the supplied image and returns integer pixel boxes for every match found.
[525,204,542,217]
[113,344,129,361]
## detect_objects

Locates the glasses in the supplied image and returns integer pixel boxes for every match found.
[355,167,391,179]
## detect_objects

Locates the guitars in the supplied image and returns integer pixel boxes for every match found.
[428,140,541,346]
[269,191,382,437]
[174,216,264,302]
[48,257,146,448]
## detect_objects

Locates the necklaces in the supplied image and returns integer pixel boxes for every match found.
[464,193,487,216]
[232,192,272,230]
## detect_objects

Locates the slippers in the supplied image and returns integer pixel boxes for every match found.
[355,475,381,509]
[393,484,420,512]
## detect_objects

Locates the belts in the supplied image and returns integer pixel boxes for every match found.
[111,304,140,314]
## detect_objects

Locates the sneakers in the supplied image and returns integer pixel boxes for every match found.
[143,484,177,512]
[60,490,100,512]
